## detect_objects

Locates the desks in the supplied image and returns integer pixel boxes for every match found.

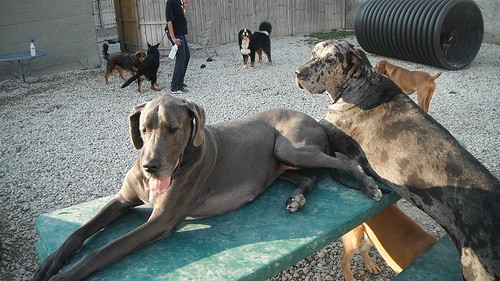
[34,170,402,281]
[0,50,47,90]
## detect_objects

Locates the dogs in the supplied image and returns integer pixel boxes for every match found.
[238,21,272,69]
[340,204,438,281]
[440,29,458,58]
[374,60,442,113]
[121,42,162,93]
[102,43,147,85]
[33,92,383,281]
[294,38,500,281]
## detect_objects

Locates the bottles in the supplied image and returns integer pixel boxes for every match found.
[30,43,36,56]
[168,43,178,59]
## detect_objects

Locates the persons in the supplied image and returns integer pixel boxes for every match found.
[164,1,191,94]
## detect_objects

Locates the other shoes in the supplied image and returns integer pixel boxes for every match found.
[172,88,187,94]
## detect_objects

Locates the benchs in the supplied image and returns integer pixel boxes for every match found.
[391,232,466,281]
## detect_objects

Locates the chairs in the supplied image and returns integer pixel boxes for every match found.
[188,20,218,60]
